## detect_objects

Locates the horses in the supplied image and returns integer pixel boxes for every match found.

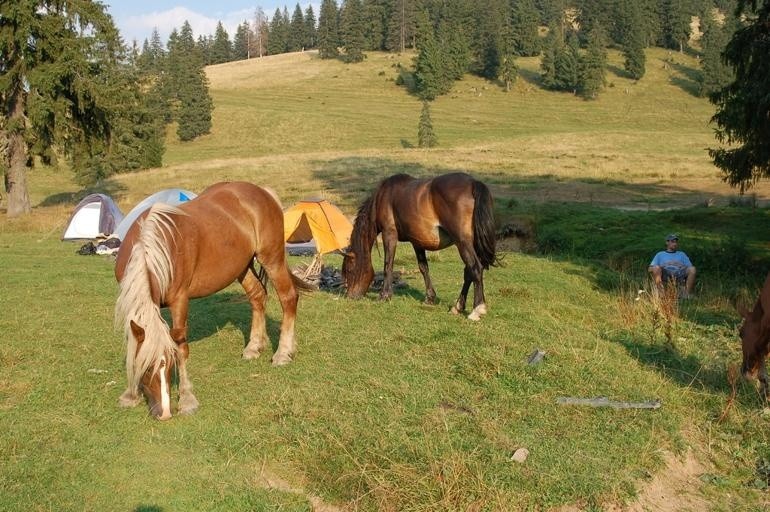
[114,180,300,422]
[737,276,770,392]
[342,172,497,322]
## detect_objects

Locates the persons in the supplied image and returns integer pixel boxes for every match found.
[646,234,697,291]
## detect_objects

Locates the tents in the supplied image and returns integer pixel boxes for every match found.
[108,188,198,243]
[278,196,355,251]
[58,192,126,241]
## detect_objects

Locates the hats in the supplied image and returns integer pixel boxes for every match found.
[664,233,679,241]
